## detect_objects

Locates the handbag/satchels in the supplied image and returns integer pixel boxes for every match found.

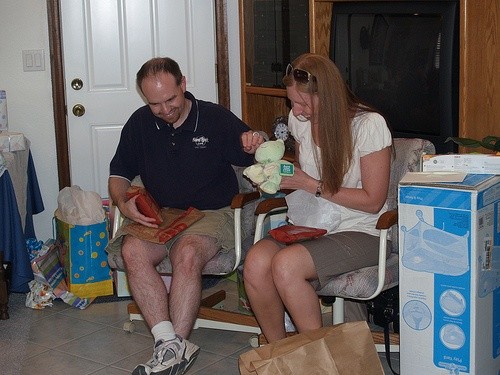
[52,207,114,298]
[238,321,385,375]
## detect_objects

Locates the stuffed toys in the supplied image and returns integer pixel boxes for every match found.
[243,139,293,194]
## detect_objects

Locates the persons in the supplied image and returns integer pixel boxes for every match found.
[244,54,393,342]
[108,58,264,374]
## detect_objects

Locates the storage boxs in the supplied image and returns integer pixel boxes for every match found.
[398,151,500,375]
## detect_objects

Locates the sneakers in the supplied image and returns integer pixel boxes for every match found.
[129,334,201,375]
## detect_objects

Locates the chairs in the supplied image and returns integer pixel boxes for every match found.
[111,136,435,353]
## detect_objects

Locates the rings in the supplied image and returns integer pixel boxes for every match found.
[253,132,259,136]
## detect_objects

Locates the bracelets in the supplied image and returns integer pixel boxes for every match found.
[315,181,323,197]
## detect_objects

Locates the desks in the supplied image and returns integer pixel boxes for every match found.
[0,131,45,320]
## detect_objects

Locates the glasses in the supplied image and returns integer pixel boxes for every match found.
[286,64,317,83]
